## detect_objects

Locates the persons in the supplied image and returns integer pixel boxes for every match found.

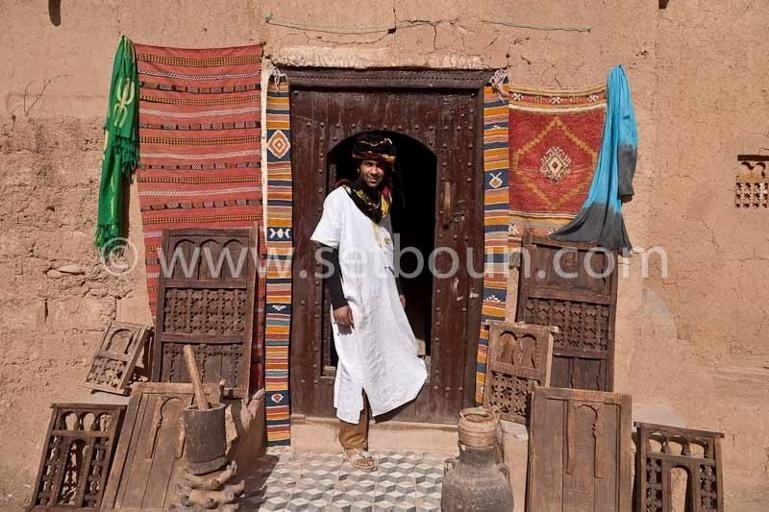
[308,136,430,474]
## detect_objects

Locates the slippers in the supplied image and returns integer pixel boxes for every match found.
[341,447,374,469]
